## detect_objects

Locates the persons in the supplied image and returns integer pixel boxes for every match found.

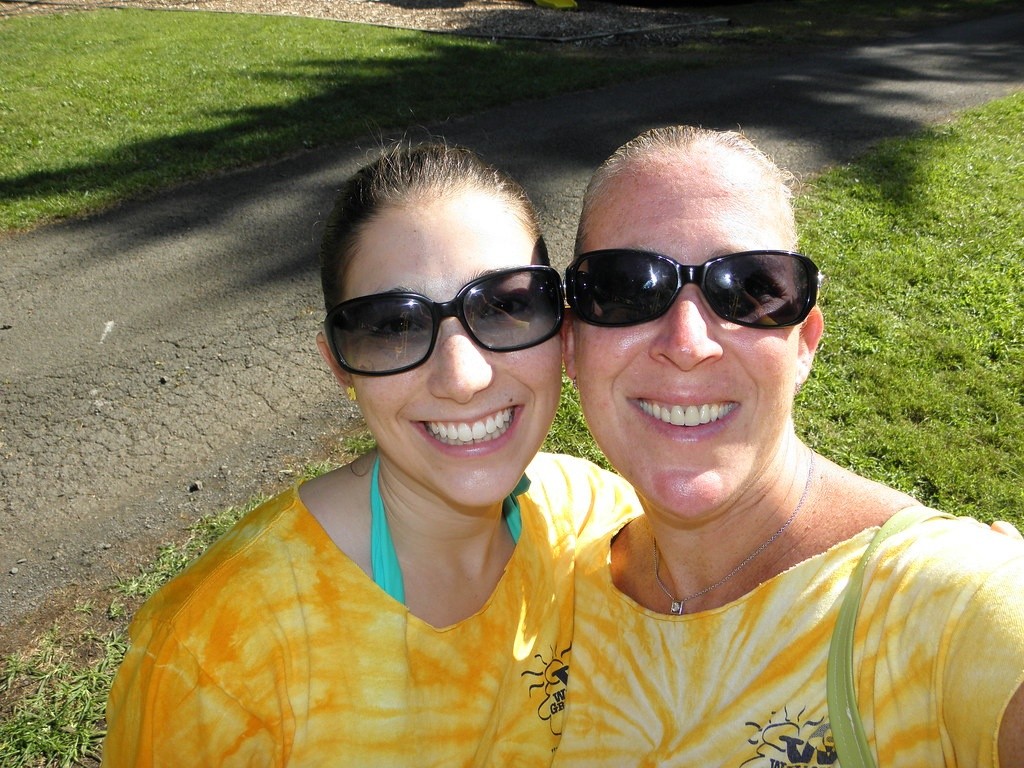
[104,143,1024,768]
[548,125,1023,768]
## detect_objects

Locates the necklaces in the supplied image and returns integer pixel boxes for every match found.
[654,444,814,615]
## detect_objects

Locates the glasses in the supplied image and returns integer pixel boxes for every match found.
[567,249,819,329]
[323,263,565,378]
